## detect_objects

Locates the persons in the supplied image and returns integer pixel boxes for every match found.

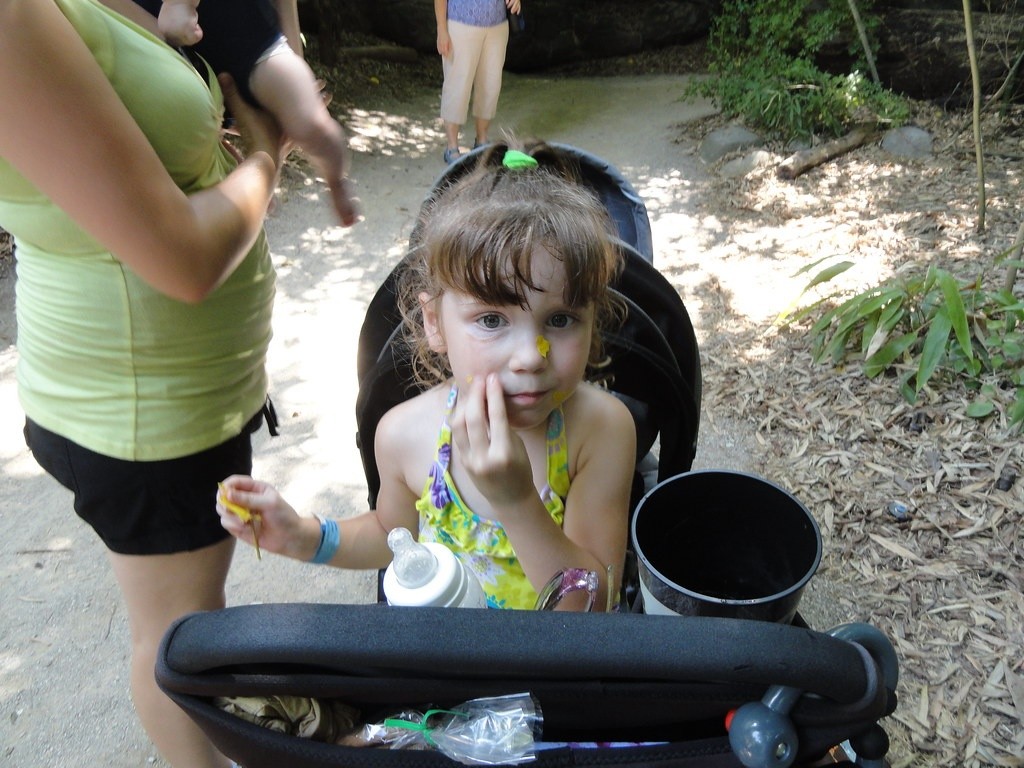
[0,0,329,768]
[434,0,521,167]
[157,0,358,225]
[215,135,638,614]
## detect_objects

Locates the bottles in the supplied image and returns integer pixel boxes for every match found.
[383,528,489,608]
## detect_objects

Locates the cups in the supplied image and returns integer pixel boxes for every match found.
[629,468,824,619]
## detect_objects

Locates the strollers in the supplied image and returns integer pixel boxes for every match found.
[155,141,905,768]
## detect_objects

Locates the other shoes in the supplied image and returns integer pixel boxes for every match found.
[443,146,461,165]
[474,137,491,149]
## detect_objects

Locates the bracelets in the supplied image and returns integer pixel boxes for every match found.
[309,514,340,565]
